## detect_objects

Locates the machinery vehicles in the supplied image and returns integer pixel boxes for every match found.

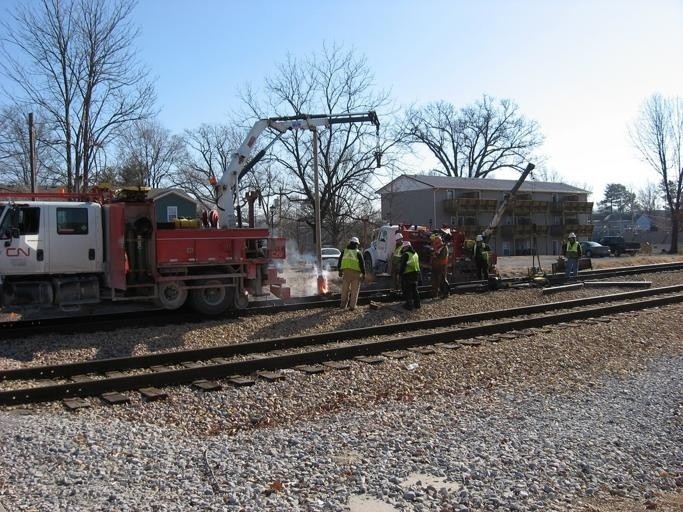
[0,109,381,318]
[361,161,535,287]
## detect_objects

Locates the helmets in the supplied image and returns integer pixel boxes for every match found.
[350,236,360,245]
[476,235,483,241]
[395,233,411,248]
[567,232,576,238]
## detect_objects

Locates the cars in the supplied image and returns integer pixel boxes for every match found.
[578,240,610,258]
[311,247,341,269]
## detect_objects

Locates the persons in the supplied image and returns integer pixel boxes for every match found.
[429,235,451,299]
[399,244,421,311]
[560,231,582,281]
[472,234,494,282]
[389,232,407,290]
[336,236,365,310]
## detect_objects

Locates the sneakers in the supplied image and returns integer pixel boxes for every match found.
[403,302,420,311]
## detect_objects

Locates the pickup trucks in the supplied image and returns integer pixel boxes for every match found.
[598,236,640,257]
